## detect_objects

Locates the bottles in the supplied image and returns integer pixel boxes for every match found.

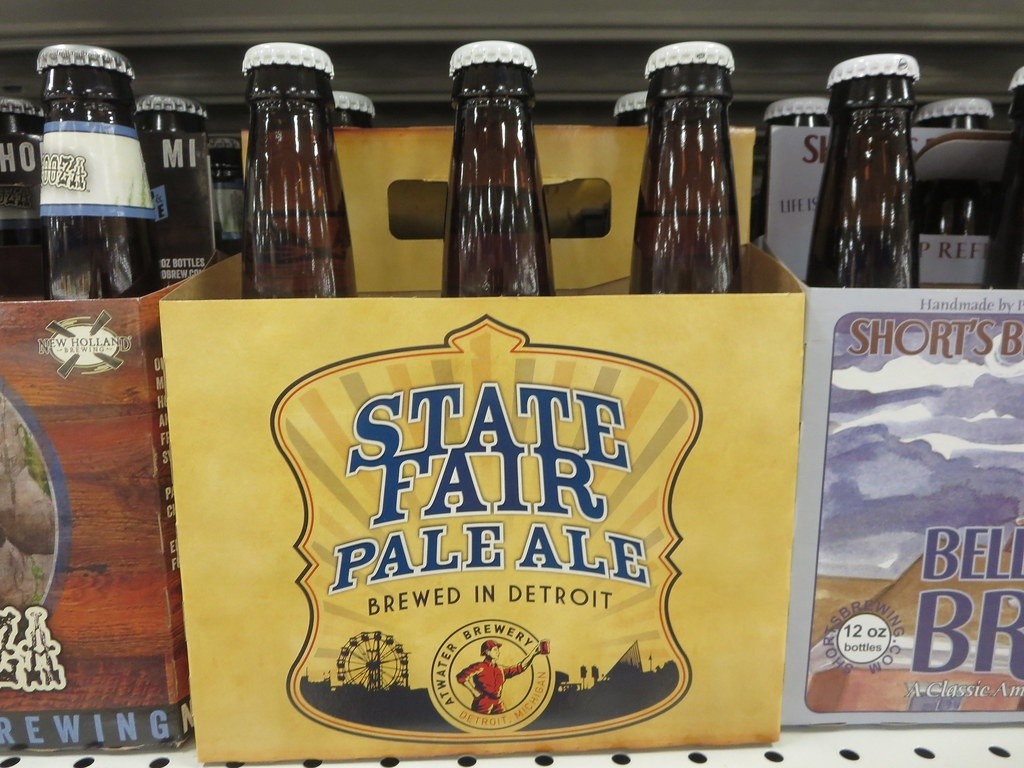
[332,91,375,127]
[0,99,51,302]
[762,98,830,285]
[208,139,246,254]
[35,44,158,299]
[918,98,996,287]
[440,40,554,298]
[134,95,214,287]
[629,41,743,294]
[990,66,1024,290]
[242,42,358,298]
[613,91,649,127]
